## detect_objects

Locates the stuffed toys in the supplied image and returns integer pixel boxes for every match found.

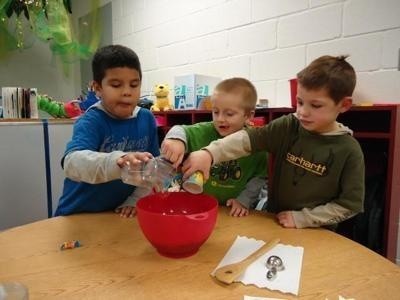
[148,80,173,112]
[35,92,82,119]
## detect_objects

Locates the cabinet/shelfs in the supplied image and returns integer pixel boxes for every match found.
[152,103,399,260]
[0,120,76,233]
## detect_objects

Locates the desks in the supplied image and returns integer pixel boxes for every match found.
[0,205,400,300]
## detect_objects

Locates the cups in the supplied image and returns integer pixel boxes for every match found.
[121,158,204,196]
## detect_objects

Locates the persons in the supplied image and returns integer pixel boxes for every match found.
[51,43,161,218]
[161,76,271,217]
[180,53,366,232]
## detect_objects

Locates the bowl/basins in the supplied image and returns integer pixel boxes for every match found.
[135,192,219,260]
